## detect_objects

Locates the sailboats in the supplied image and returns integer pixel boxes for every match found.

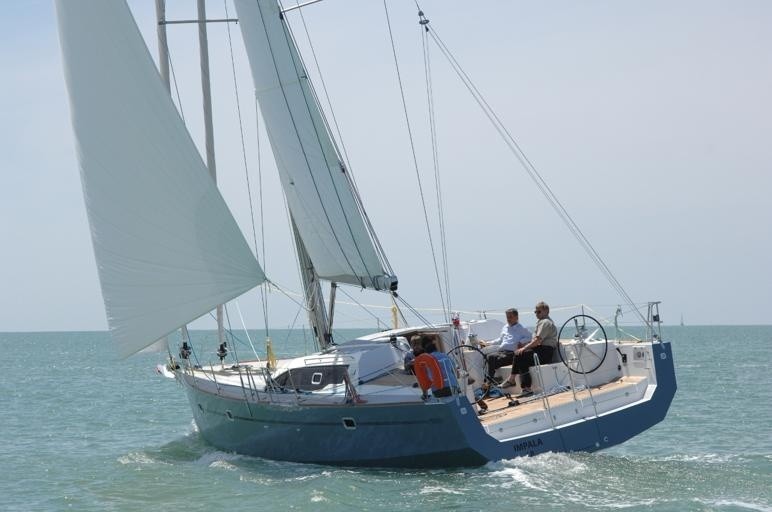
[56,1,682,466]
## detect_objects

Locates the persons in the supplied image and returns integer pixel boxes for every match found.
[479,308,533,400]
[421,337,488,411]
[497,301,558,399]
[405,335,423,375]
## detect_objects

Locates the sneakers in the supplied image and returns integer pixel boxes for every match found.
[496,379,517,388]
[515,389,533,399]
